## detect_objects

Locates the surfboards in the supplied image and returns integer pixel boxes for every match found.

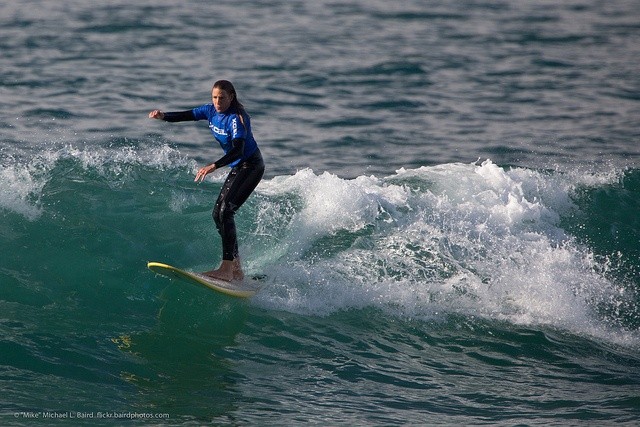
[147,262,255,297]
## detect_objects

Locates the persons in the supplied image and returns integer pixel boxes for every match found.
[148,78,267,282]
